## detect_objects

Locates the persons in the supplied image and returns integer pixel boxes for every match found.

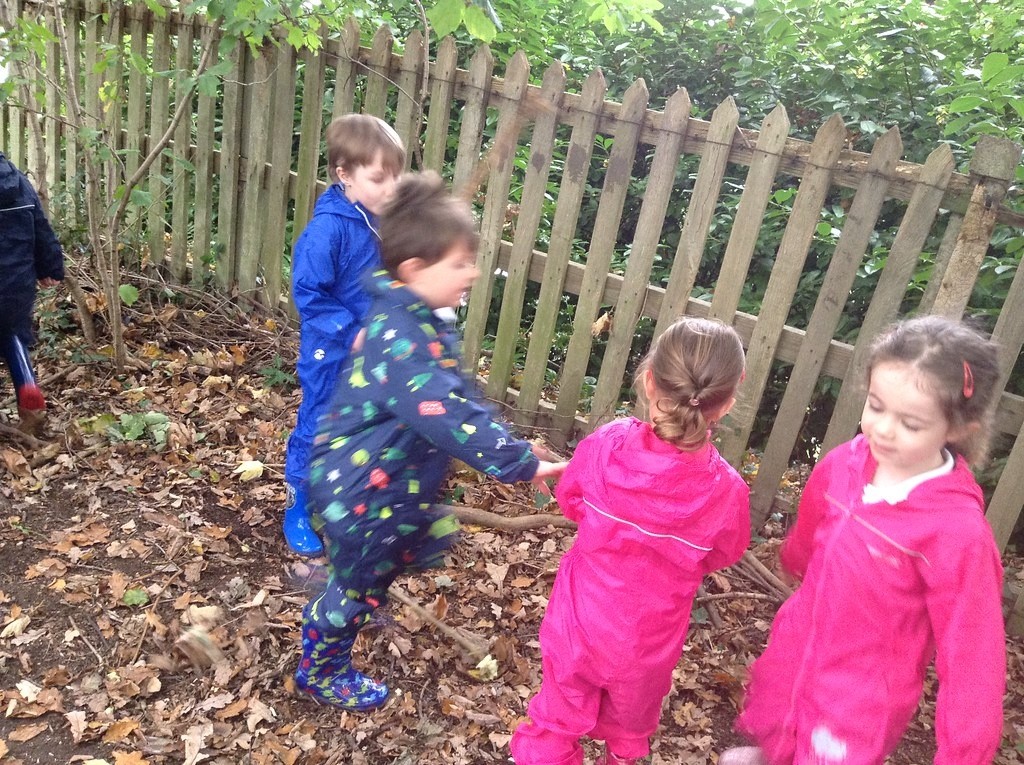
[295,170,570,711]
[0,150,65,420]
[734,315,1006,765]
[509,317,751,765]
[282,114,407,559]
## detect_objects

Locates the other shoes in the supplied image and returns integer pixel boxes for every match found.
[595,747,636,765]
[18,384,47,421]
[718,746,764,765]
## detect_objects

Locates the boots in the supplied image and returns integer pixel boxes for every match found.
[294,603,389,710]
[283,481,323,558]
